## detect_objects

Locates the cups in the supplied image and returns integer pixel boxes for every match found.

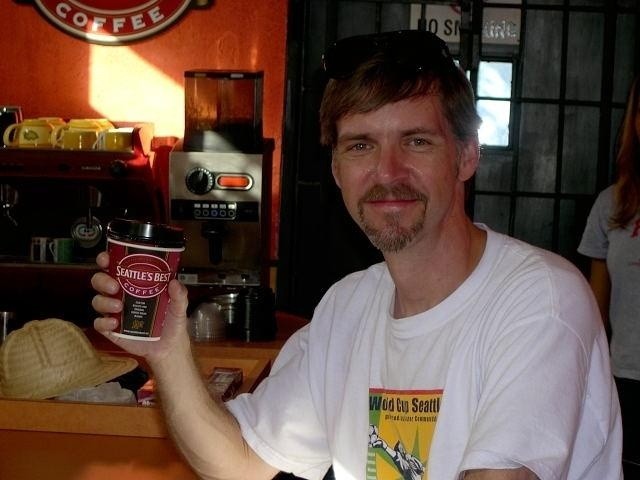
[189,287,274,346]
[0,105,136,151]
[48,238,75,264]
[100,217,189,342]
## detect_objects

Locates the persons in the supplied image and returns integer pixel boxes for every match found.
[576,80,639,478]
[90,29,627,479]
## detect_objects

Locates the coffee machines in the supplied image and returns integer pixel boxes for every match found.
[169,69,277,314]
[0,151,146,295]
